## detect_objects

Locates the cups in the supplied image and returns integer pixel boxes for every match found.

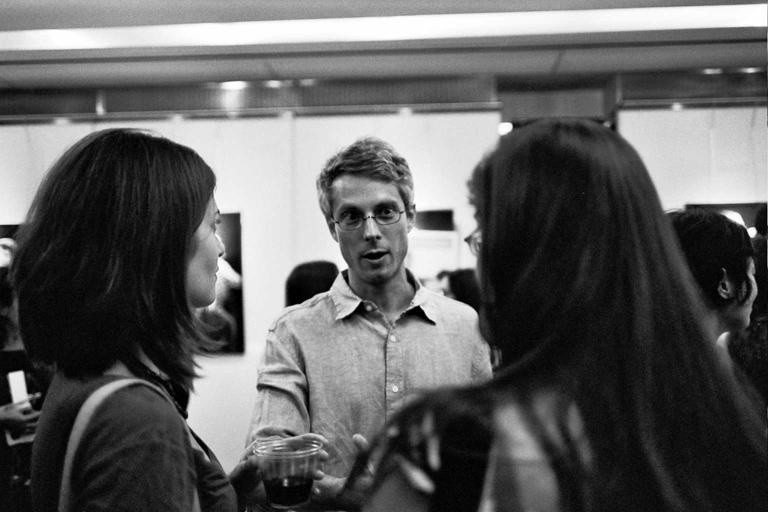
[250,434,324,511]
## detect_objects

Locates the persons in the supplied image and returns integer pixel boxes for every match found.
[361,116,768,510]
[438,269,480,311]
[665,210,759,343]
[244,135,496,512]
[716,235,767,426]
[285,261,337,308]
[1,265,56,512]
[7,128,331,510]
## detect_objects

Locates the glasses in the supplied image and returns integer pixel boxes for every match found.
[328,206,410,233]
[461,223,483,257]
